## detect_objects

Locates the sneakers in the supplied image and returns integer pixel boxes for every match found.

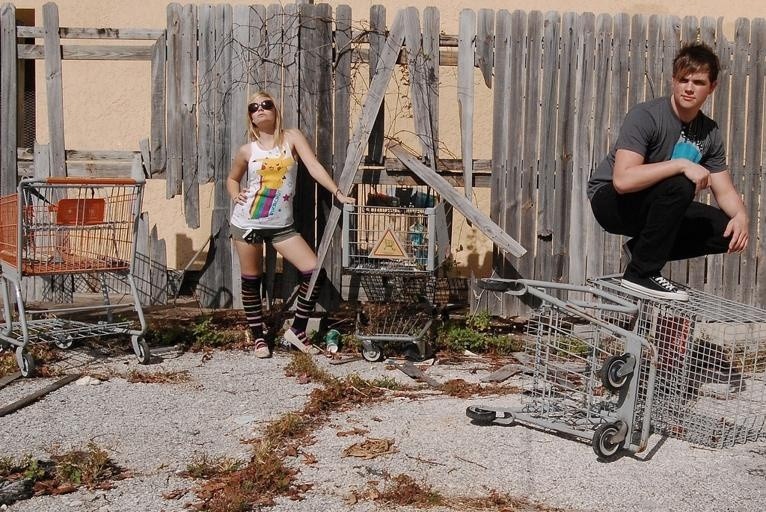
[620,238,690,303]
[254,338,271,359]
[284,326,321,355]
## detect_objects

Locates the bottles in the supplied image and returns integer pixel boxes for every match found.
[408,218,427,266]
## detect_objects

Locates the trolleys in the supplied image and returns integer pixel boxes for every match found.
[334,184,460,361]
[460,267,765,458]
[0,173,158,379]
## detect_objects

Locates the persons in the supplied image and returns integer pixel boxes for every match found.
[225,90,357,360]
[587,43,750,303]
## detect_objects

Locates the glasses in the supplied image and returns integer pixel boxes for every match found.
[248,100,274,113]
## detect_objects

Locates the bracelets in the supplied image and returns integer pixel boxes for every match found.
[334,189,343,198]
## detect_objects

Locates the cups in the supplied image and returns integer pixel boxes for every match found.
[412,191,436,208]
[323,330,342,353]
